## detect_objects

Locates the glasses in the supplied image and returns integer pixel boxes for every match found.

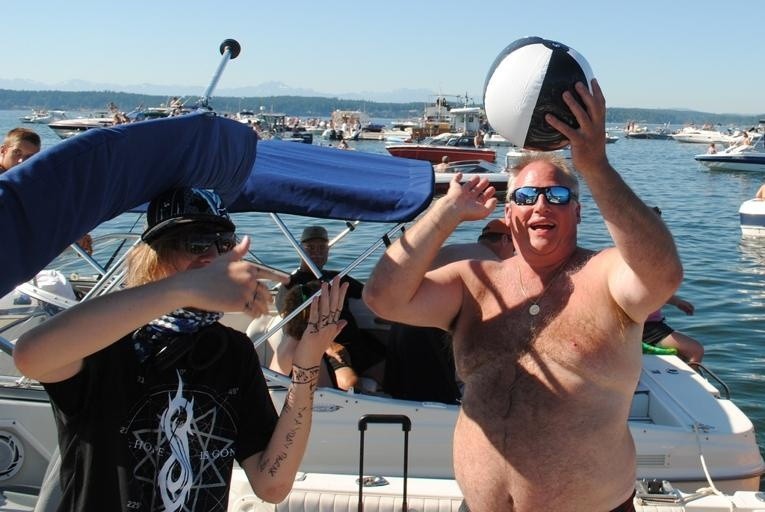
[510,186,576,204]
[163,227,236,255]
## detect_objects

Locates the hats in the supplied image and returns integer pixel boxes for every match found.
[301,227,328,242]
[141,186,235,250]
[481,220,512,234]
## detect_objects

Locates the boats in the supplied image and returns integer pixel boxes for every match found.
[0,38,765,511]
[624,120,670,140]
[694,119,765,173]
[738,198,765,235]
[666,124,741,144]
[429,159,512,194]
[384,131,495,166]
[19,108,66,125]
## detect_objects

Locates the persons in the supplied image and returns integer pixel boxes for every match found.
[107,94,361,150]
[361,78,685,512]
[473,129,483,148]
[389,216,513,405]
[625,120,765,155]
[642,207,705,370]
[756,183,765,200]
[276,225,390,391]
[11,186,350,512]
[0,127,94,301]
[432,154,455,174]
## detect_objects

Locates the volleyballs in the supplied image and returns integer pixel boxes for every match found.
[484,37,594,150]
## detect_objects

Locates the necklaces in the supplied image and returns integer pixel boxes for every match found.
[515,257,570,315]
